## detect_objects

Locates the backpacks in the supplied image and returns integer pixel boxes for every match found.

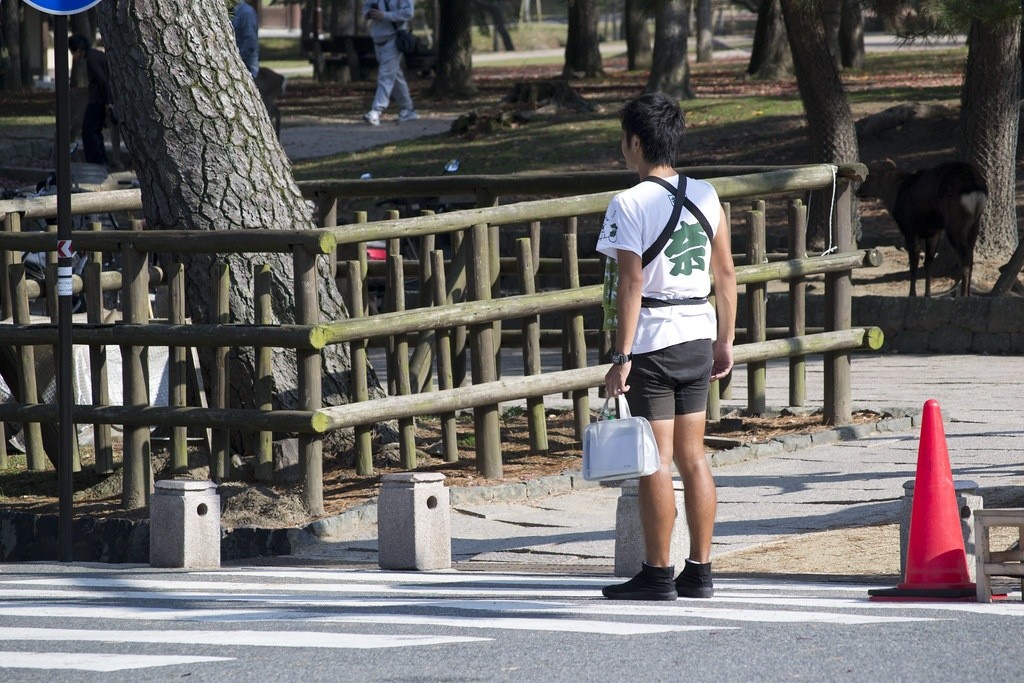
[20,176,97,282]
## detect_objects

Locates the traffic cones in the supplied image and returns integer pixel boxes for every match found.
[867,398,1008,603]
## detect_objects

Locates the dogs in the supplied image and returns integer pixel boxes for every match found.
[855,161,988,300]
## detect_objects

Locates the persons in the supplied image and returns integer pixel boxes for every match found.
[225,0,260,80]
[68,34,117,166]
[594,90,738,602]
[361,1,419,128]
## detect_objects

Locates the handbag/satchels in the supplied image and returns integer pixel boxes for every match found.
[396,34,416,52]
[581,394,660,482]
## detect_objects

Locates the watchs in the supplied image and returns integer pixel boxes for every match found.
[611,350,633,364]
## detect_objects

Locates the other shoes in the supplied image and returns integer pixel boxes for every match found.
[363,110,379,126]
[674,558,714,598]
[398,109,416,121]
[602,561,678,600]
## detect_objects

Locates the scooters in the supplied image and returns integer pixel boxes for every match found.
[343,157,462,312]
[0,140,85,315]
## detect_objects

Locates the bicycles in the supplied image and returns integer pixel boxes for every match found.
[21,180,157,315]
